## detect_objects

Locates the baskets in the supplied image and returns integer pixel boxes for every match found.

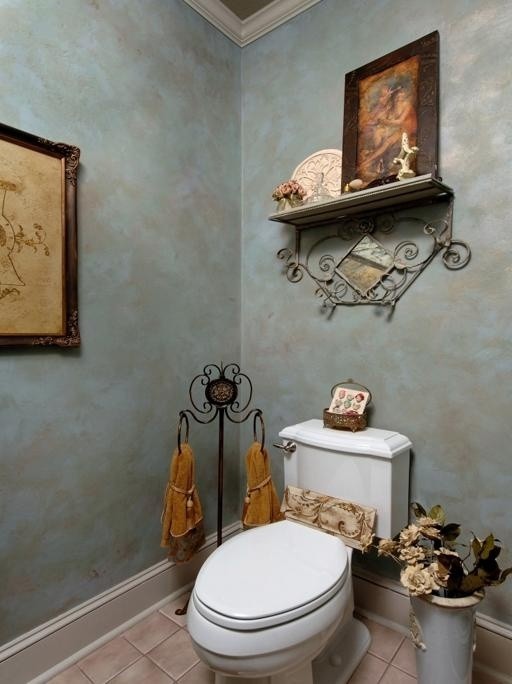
[323,408,367,433]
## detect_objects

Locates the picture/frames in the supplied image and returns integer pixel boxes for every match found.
[341,30,440,193]
[0,123,80,350]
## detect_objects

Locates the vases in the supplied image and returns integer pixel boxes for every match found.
[277,198,302,212]
[408,587,485,684]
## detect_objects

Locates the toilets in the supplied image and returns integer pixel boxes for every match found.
[188,420,412,684]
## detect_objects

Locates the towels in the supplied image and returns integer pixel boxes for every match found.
[242,443,284,531]
[160,444,206,564]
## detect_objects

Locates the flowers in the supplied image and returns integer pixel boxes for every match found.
[377,502,512,597]
[273,180,307,202]
